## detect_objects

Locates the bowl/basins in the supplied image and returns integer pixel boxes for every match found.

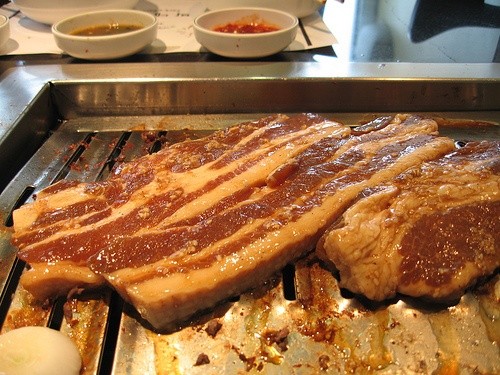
[51,7,158,63]
[0,12,11,46]
[13,1,141,28]
[192,5,300,59]
[204,0,324,19]
